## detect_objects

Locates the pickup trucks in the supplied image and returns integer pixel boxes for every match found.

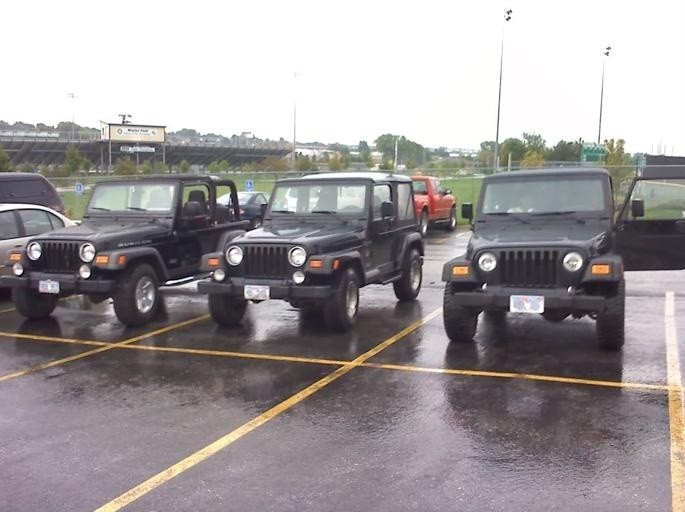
[398,176,458,239]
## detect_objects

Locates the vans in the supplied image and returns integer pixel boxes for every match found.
[283,173,394,211]
[0,171,68,218]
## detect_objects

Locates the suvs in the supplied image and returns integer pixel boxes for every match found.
[441,168,685,349]
[195,169,429,335]
[1,170,255,328]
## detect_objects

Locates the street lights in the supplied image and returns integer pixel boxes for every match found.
[596,45,611,142]
[493,7,514,173]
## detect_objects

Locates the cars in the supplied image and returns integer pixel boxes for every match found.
[214,191,272,230]
[0,203,81,287]
